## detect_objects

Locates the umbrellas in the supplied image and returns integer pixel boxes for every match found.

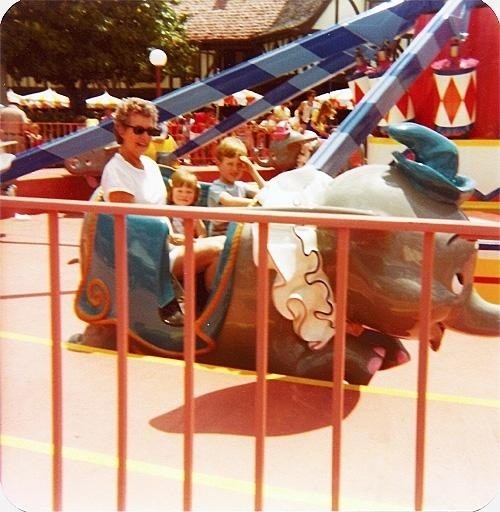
[85,89,123,109]
[19,87,71,110]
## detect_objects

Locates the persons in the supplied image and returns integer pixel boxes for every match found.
[101,96,227,293]
[206,135,267,237]
[0,93,29,155]
[30,123,43,147]
[150,90,339,166]
[166,168,209,240]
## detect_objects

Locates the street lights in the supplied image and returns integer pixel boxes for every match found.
[147,48,169,99]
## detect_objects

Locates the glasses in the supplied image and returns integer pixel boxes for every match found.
[123,122,154,136]
